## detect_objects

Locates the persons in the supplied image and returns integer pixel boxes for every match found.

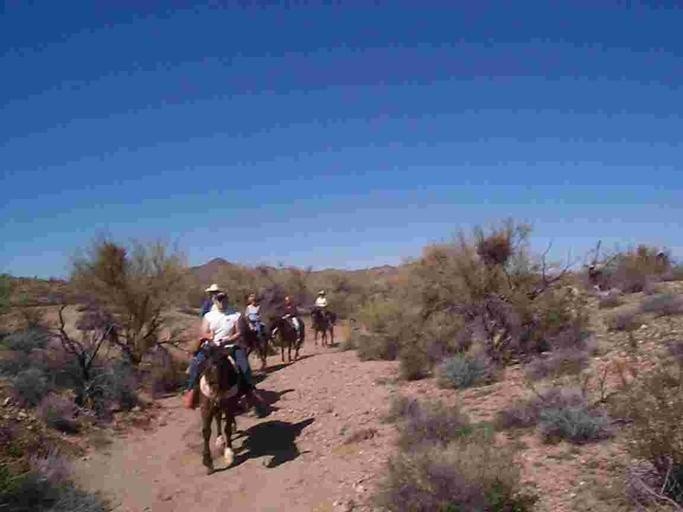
[244,293,265,348]
[271,295,301,343]
[181,292,262,408]
[183,282,222,376]
[309,289,336,329]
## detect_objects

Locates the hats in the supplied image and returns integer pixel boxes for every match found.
[203,283,225,292]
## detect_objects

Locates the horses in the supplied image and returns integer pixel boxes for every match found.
[197,346,247,475]
[240,322,271,371]
[309,307,336,349]
[268,316,305,363]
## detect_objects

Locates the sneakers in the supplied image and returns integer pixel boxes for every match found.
[183,389,196,409]
[246,390,266,407]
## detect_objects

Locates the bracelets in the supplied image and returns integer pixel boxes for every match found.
[220,338,232,346]
